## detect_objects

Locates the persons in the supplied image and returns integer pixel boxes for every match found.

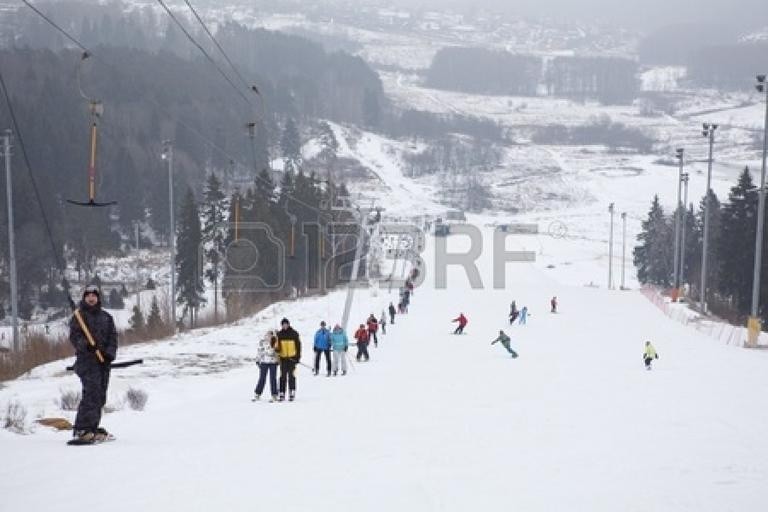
[269,316,302,401]
[509,299,519,324]
[355,323,368,363]
[452,312,468,336]
[64,284,118,444]
[490,330,518,359]
[251,328,279,400]
[550,296,559,313]
[330,325,350,377]
[311,321,331,375]
[642,341,659,371]
[367,268,419,349]
[521,306,530,326]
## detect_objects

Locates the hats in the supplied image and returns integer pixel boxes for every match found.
[84,284,100,297]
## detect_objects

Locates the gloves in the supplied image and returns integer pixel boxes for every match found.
[100,356,110,366]
[88,342,98,352]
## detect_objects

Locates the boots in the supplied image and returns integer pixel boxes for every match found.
[252,391,295,402]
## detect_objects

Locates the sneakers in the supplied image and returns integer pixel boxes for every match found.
[74,429,108,440]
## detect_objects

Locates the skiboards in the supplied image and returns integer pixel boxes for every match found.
[250,395,278,403]
[277,391,296,402]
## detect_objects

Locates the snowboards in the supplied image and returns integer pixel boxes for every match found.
[66,433,115,446]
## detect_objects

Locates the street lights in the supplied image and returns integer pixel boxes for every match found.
[673,122,719,313]
[0,126,20,365]
[160,139,176,336]
[745,72,768,348]
[608,202,627,290]
